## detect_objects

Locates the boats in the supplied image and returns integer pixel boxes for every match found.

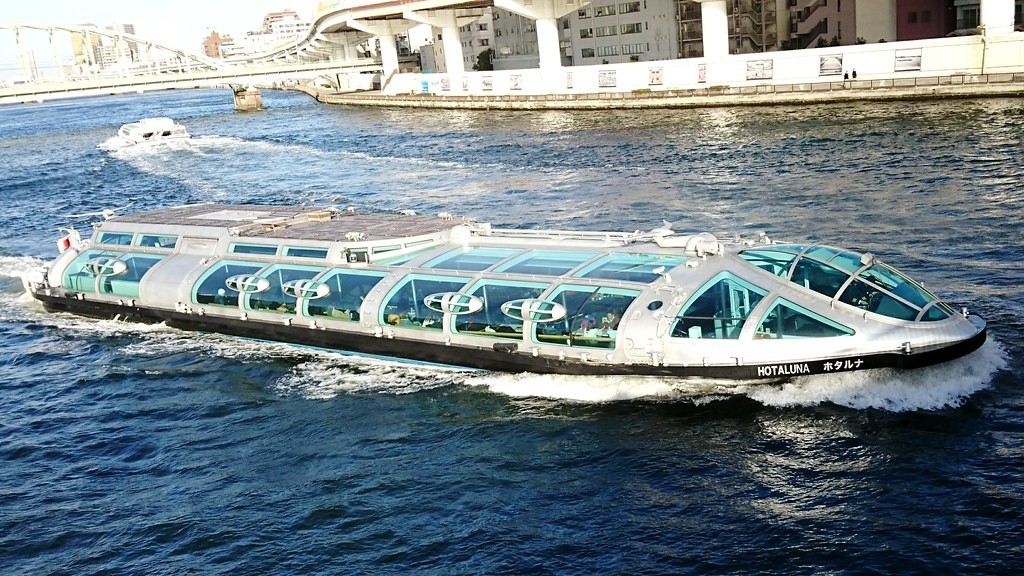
[31,202,988,381]
[234,88,263,113]
[99,113,192,152]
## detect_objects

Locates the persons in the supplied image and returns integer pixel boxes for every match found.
[213,286,620,348]
[844,275,874,307]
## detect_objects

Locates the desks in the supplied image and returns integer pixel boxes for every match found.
[572,328,611,337]
